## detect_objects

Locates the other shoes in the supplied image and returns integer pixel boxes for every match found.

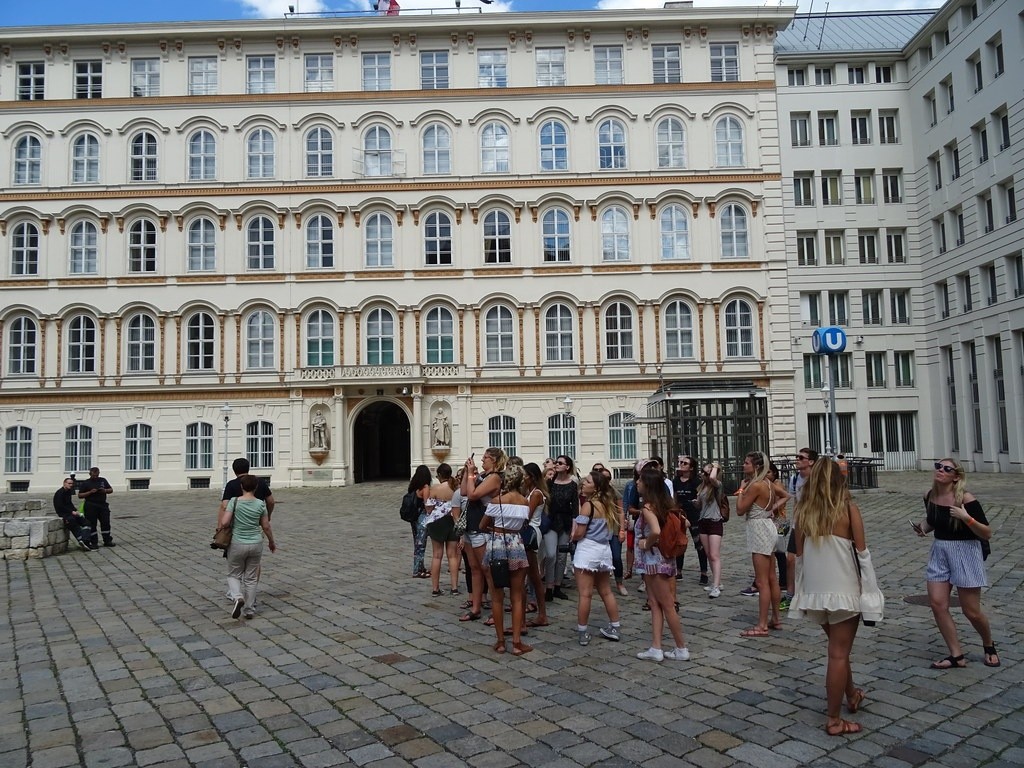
[232,597,245,618]
[638,580,646,592]
[412,567,572,654]
[709,587,721,597]
[227,591,233,599]
[616,587,628,596]
[698,574,708,584]
[244,612,254,619]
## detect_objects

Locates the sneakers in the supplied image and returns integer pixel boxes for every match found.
[741,586,760,596]
[637,647,664,662]
[600,623,621,640]
[579,630,592,645]
[664,647,690,661]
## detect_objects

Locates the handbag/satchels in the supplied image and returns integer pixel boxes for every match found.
[213,525,232,548]
[489,558,511,588]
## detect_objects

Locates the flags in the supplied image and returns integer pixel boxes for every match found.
[378,0,400,16]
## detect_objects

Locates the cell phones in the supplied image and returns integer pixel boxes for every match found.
[472,453,475,457]
[909,520,926,537]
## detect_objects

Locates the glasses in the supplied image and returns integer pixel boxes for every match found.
[796,455,809,460]
[935,462,958,473]
[555,461,566,465]
[680,460,688,465]
[701,469,708,476]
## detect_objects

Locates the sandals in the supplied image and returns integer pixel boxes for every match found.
[983,641,1000,666]
[932,654,966,668]
[847,687,865,713]
[768,621,783,631]
[741,625,769,636]
[826,718,863,736]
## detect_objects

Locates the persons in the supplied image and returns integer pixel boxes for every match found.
[912,458,1000,668]
[217,458,274,606]
[400,447,674,655]
[741,462,788,597]
[734,452,791,637]
[692,461,724,598]
[634,469,689,662]
[311,410,327,448]
[78,467,116,549]
[54,478,92,551]
[788,455,884,735]
[221,475,275,619]
[433,408,448,447]
[779,447,818,610]
[672,457,709,585]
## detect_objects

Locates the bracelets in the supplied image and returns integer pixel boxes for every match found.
[644,542,647,549]
[620,530,624,532]
[468,476,474,479]
[967,518,974,526]
[738,489,743,493]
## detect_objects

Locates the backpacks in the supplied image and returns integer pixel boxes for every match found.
[658,506,688,559]
[400,490,424,522]
[715,490,730,522]
